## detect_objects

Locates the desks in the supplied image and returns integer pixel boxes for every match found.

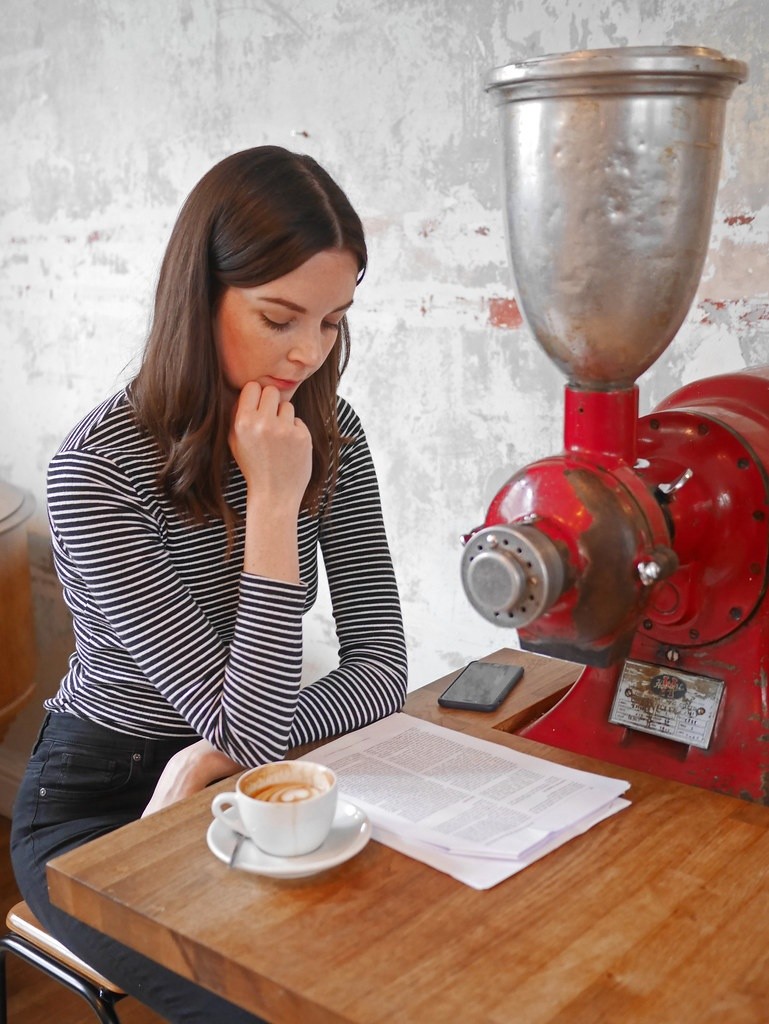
[45,646,768,1024]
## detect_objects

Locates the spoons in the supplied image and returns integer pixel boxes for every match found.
[227,830,250,871]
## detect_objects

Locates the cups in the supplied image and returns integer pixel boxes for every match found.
[211,760,339,856]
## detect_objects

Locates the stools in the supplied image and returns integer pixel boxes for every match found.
[0,901,127,1024]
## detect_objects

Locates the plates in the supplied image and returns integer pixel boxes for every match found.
[206,797,373,880]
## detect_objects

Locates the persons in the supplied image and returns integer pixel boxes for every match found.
[6,143,410,1023]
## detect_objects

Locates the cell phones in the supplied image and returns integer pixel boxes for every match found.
[437,660,523,712]
